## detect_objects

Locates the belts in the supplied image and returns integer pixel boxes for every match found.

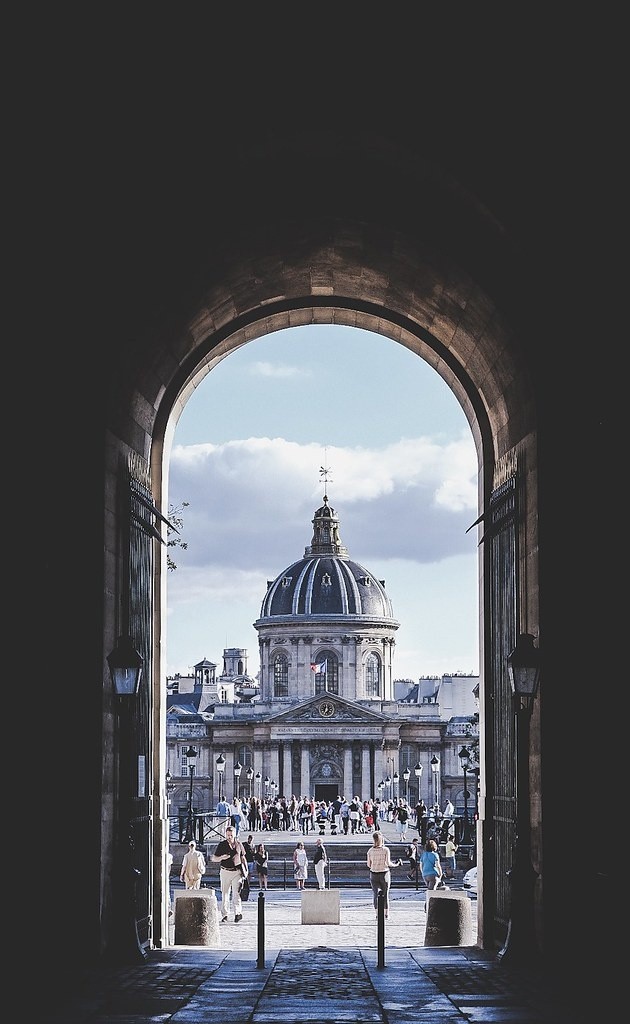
[221,867,237,871]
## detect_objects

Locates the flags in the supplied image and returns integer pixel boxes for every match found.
[311,662,325,673]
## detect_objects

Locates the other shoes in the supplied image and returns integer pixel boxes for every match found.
[222,916,227,922]
[450,876,458,880]
[296,887,302,891]
[235,914,242,923]
[442,872,447,879]
[301,887,305,890]
[406,875,415,881]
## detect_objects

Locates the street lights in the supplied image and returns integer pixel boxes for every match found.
[414,761,423,803]
[182,744,198,844]
[429,754,440,817]
[458,745,475,845]
[216,753,226,804]
[264,775,279,797]
[377,772,400,802]
[245,766,254,799]
[402,766,412,803]
[233,760,243,799]
[255,771,262,799]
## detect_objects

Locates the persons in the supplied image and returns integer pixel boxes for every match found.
[212,826,246,921]
[367,832,400,920]
[419,840,443,912]
[255,843,269,890]
[443,836,458,880]
[407,838,420,881]
[180,841,206,889]
[216,796,454,841]
[243,835,256,885]
[314,838,328,890]
[293,842,308,890]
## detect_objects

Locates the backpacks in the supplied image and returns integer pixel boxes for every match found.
[405,845,415,857]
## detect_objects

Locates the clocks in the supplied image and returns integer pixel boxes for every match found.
[318,702,334,717]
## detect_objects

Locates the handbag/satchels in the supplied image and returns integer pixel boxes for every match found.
[238,865,249,901]
[197,854,205,875]
